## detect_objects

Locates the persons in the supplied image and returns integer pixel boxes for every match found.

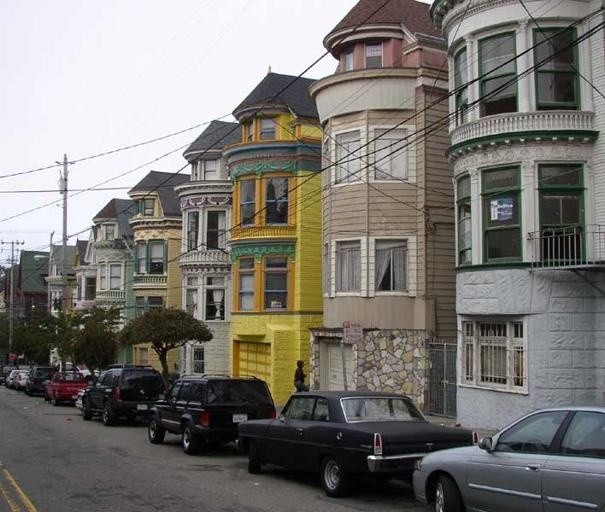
[293,360,307,393]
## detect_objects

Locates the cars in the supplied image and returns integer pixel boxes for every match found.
[11,372,30,391]
[5,370,28,388]
[45,372,88,405]
[82,364,167,425]
[147,374,277,454]
[236,390,481,498]
[412,405,605,512]
[26,366,58,397]
